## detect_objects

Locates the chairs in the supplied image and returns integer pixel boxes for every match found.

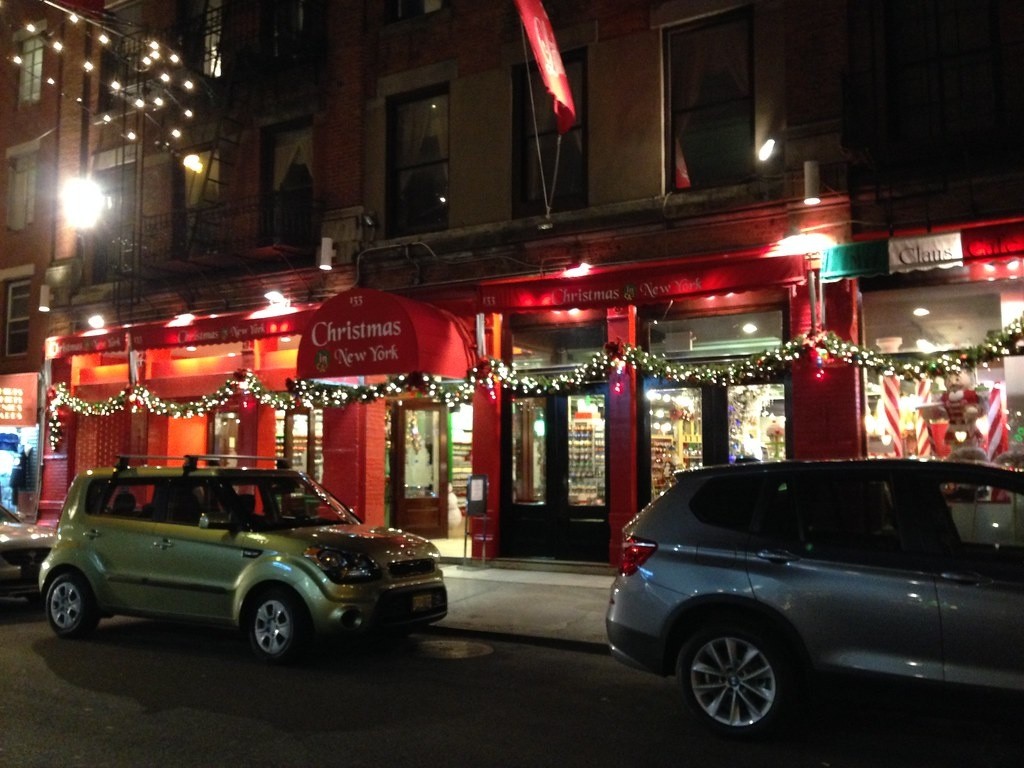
[112,492,137,515]
[230,493,263,524]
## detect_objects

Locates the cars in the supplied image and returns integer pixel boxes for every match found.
[0,506,59,609]
[603,454,1024,741]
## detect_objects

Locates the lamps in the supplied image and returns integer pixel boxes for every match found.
[38,284,54,312]
[803,160,821,205]
[319,237,336,271]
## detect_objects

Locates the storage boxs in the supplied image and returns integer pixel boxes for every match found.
[574,412,602,420]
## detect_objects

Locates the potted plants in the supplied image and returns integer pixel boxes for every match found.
[17,446,37,516]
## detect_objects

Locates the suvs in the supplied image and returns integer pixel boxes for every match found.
[37,454,449,667]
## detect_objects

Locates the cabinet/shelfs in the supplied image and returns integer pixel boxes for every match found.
[569,417,603,505]
[274,408,324,483]
[453,439,471,514]
[653,416,702,498]
